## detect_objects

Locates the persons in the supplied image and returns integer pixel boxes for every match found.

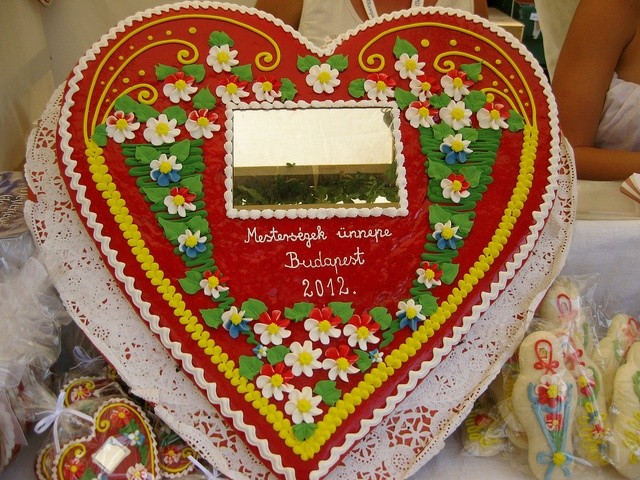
[253,0,490,57]
[550,0,640,182]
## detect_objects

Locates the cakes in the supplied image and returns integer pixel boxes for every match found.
[54,0,562,480]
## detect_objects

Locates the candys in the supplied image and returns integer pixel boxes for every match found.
[457,275,640,480]
[32,331,224,479]
